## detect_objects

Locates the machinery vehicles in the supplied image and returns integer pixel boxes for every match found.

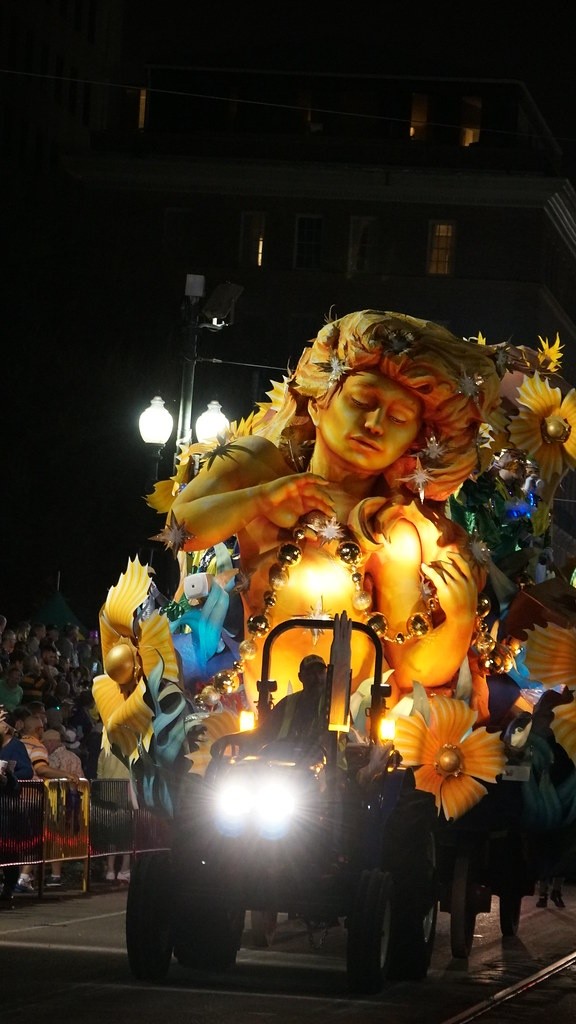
[124,619,447,994]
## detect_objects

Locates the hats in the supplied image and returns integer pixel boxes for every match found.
[300,655,328,674]
[62,730,81,749]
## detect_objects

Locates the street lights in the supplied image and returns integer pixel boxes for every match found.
[139,334,230,574]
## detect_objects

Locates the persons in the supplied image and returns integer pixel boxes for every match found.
[210,654,354,764]
[163,310,476,730]
[0,615,141,899]
[447,673,576,969]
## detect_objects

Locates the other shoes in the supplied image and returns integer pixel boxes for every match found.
[105,871,116,885]
[45,876,62,887]
[550,890,565,907]
[15,879,34,893]
[117,871,131,884]
[536,893,549,908]
